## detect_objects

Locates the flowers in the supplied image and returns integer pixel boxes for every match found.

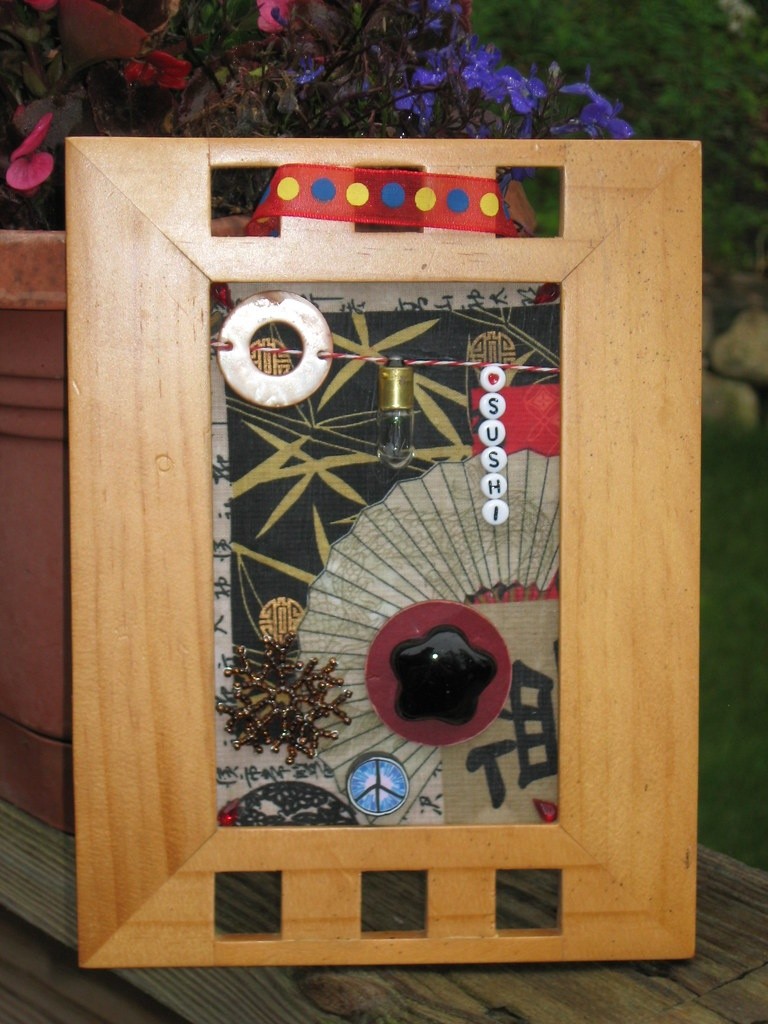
[0,0,641,224]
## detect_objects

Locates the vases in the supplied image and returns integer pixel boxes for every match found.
[0,217,277,826]
